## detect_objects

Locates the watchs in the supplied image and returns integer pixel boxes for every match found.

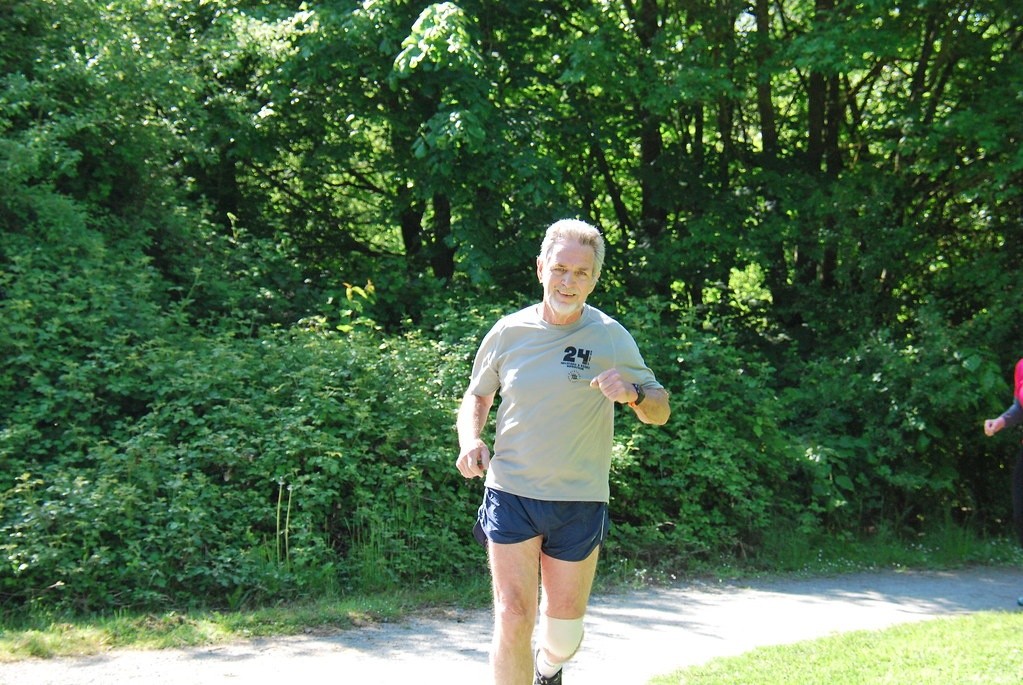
[629,384,645,407]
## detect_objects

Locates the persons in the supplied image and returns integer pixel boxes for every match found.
[455,218,671,685]
[985,359,1023,607]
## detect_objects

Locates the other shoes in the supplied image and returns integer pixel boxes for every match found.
[534,649,562,685]
